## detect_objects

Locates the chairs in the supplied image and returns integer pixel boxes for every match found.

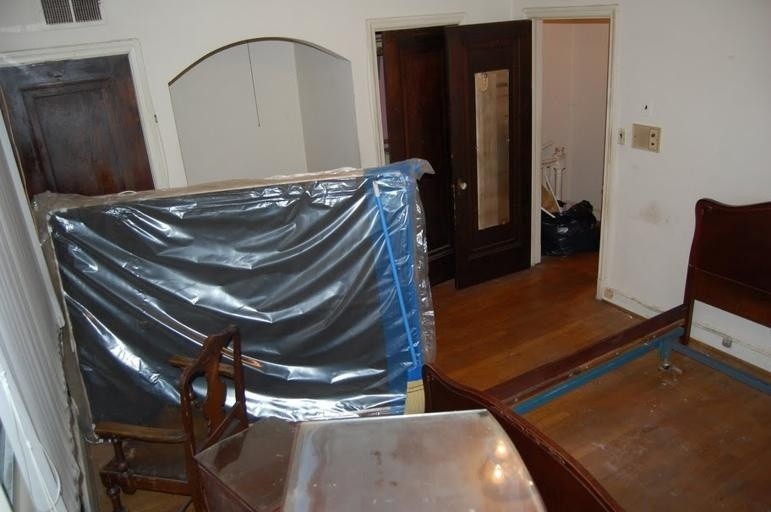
[94,323,250,511]
